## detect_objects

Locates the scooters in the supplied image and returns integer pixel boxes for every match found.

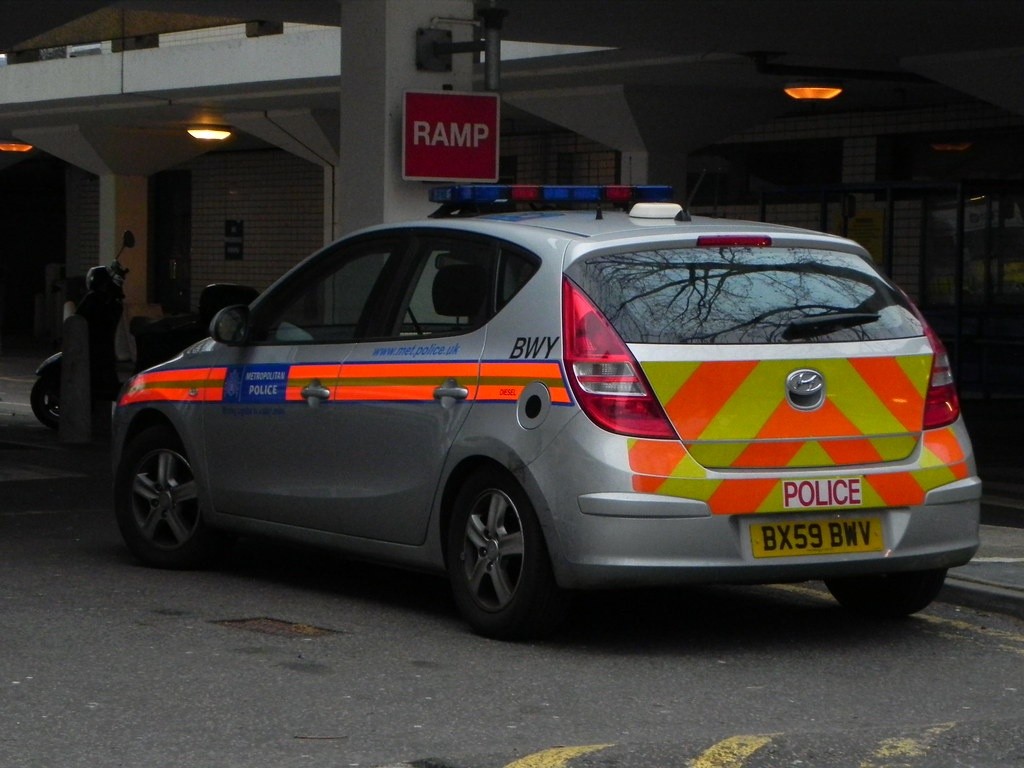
[31,229,265,441]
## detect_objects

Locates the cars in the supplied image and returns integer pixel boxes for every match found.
[110,180,982,639]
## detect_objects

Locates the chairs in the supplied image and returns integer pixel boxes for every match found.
[432,263,490,329]
